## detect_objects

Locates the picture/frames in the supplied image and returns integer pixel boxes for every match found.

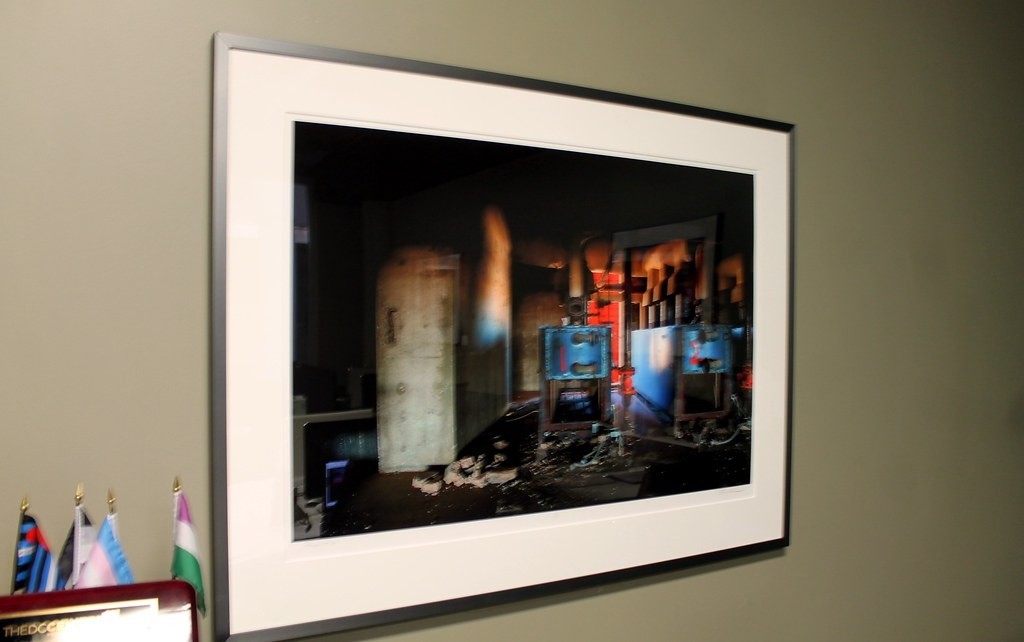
[0,579,200,642]
[208,30,797,642]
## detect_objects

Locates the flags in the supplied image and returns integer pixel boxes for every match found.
[13,515,54,593]
[75,516,134,588]
[54,512,96,591]
[173,493,206,616]
